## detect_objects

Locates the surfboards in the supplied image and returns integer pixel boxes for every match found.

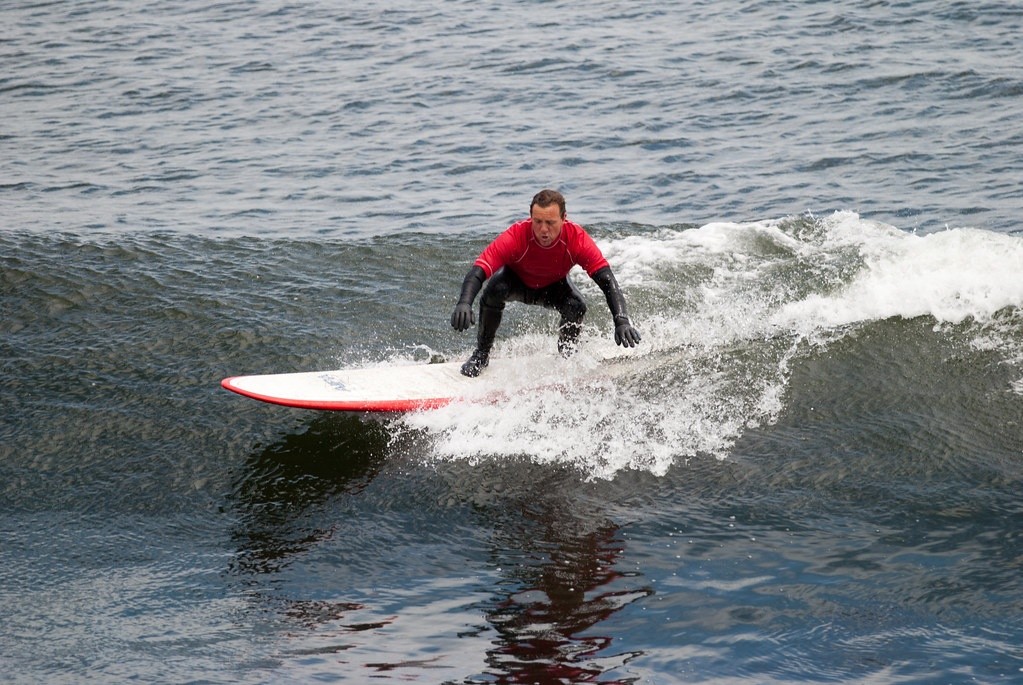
[220,350,601,412]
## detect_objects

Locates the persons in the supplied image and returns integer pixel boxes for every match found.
[449,189,641,377]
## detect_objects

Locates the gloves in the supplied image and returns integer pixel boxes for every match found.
[613,313,642,348]
[450,304,476,332]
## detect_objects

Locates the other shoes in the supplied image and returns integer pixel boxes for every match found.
[557,340,577,359]
[460,349,490,377]
